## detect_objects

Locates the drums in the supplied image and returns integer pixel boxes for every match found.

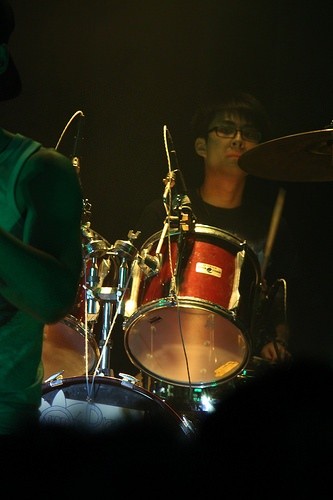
[40,376,192,439]
[122,224,261,389]
[38,224,121,387]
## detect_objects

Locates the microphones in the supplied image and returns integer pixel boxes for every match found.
[68,114,85,175]
[257,278,281,313]
[165,129,192,217]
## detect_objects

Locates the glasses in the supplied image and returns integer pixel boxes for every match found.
[205,125,263,143]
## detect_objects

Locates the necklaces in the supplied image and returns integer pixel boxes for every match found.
[197,183,213,219]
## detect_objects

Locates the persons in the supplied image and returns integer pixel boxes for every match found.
[0,125,85,500]
[109,89,300,431]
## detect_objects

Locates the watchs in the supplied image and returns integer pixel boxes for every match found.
[275,339,289,347]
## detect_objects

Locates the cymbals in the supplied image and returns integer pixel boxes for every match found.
[238,125,333,183]
